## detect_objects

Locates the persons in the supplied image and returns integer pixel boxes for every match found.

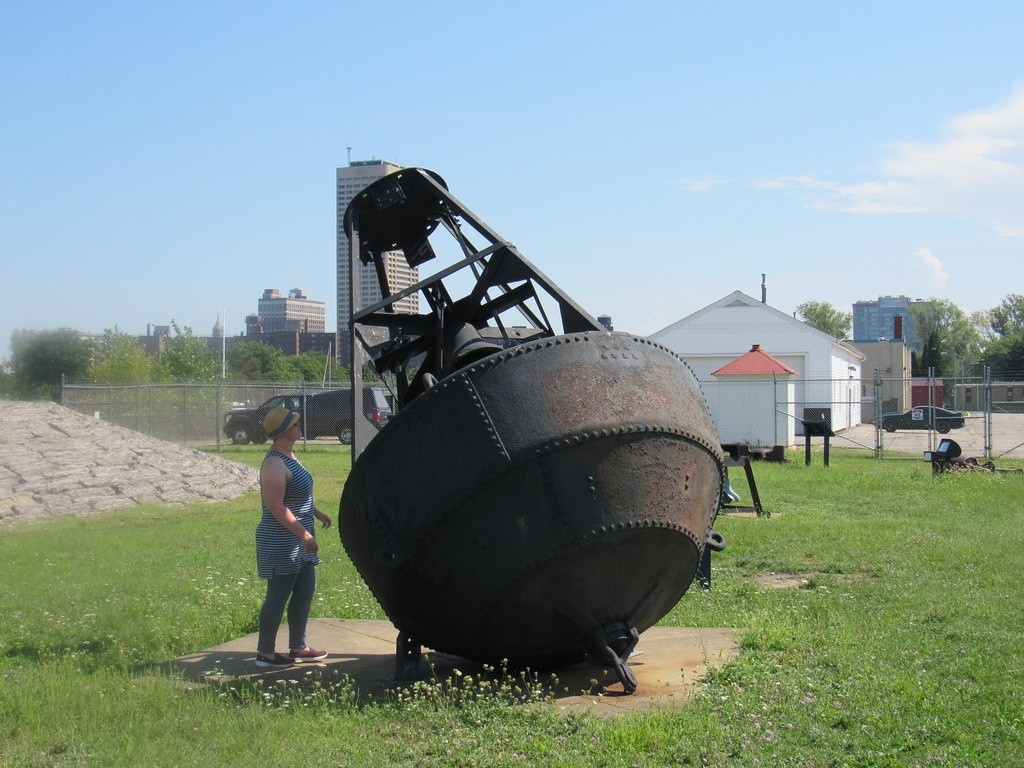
[255,406,333,668]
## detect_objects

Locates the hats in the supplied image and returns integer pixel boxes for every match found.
[263,407,300,440]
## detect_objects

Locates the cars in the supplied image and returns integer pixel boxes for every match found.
[872,406,965,435]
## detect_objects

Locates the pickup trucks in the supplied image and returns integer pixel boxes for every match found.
[225,384,391,446]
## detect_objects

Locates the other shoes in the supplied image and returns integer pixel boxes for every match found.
[255,653,295,667]
[288,646,328,663]
